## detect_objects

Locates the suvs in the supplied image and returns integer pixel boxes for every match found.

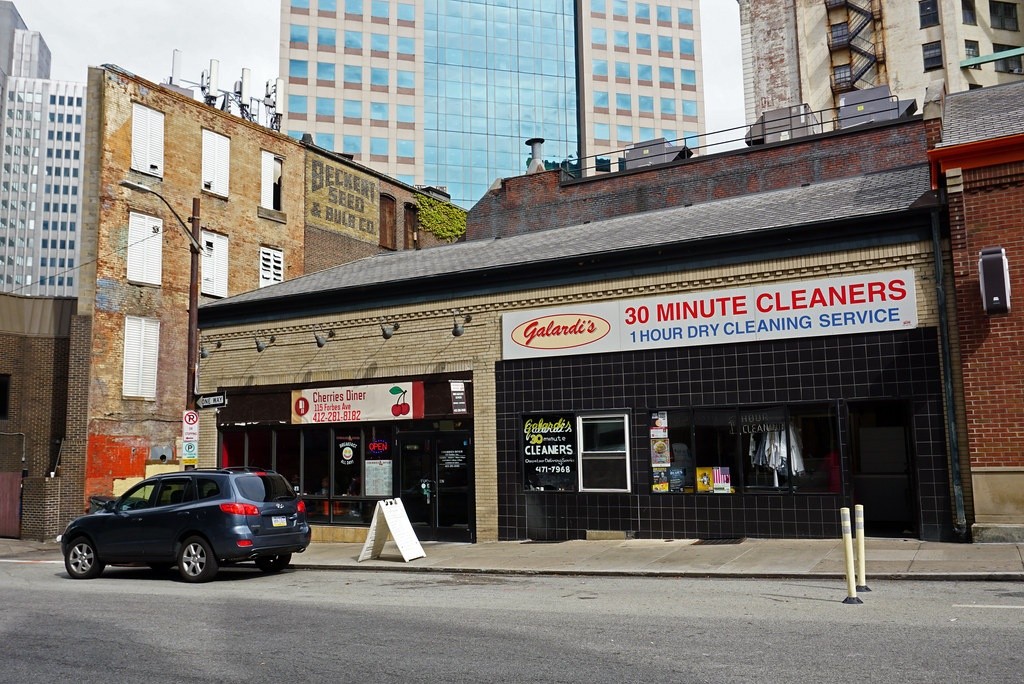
[60,466,312,584]
[360,458,473,527]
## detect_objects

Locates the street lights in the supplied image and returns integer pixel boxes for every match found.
[121,181,201,472]
